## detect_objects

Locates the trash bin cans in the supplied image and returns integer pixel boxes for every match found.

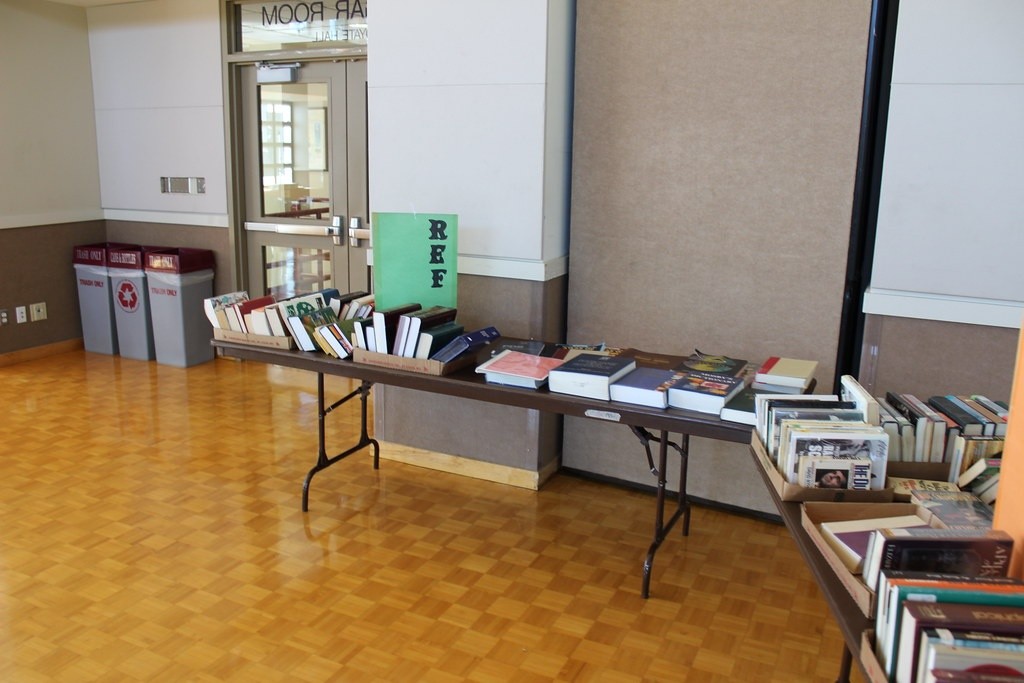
[144,246,217,369]
[107,244,175,362]
[73,241,127,356]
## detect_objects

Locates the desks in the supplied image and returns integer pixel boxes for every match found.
[748,445,951,683]
[207,330,754,599]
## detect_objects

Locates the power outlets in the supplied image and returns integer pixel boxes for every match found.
[16,306,28,324]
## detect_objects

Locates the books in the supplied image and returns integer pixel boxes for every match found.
[373,303,422,354]
[755,375,1024,683]
[403,306,457,358]
[414,324,464,360]
[204,288,375,359]
[431,327,500,363]
[353,317,373,351]
[366,325,377,352]
[475,339,819,426]
[392,305,445,357]
[351,332,358,348]
